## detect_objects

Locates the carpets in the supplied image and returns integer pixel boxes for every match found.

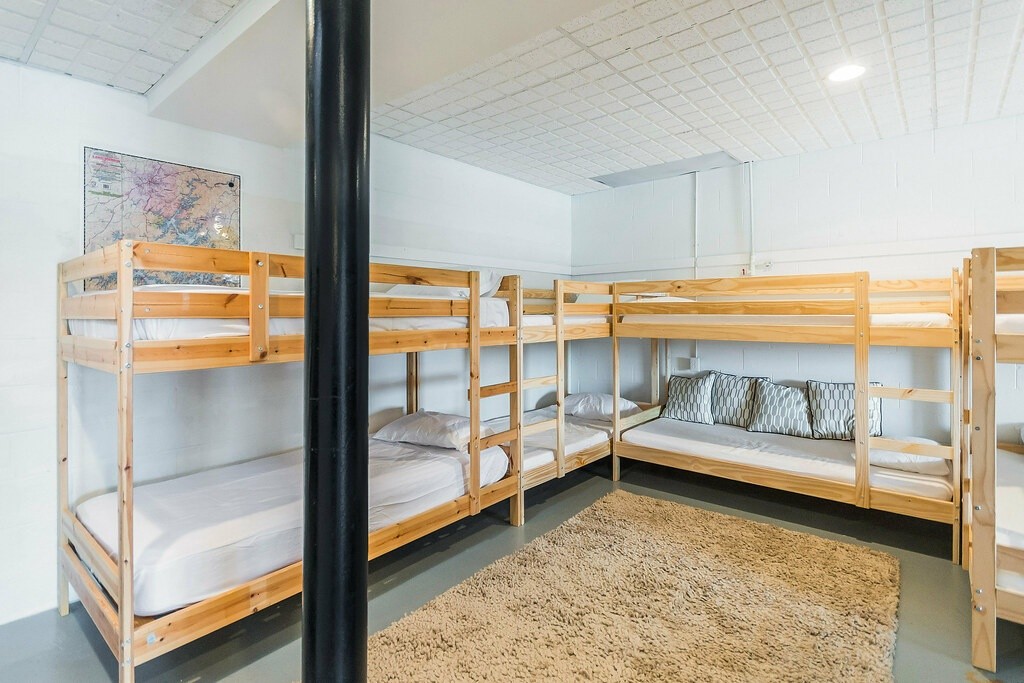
[367,489,900,683]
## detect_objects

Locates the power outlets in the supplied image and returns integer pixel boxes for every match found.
[690,358,700,371]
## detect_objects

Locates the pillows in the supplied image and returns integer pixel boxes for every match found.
[806,380,882,441]
[386,263,503,300]
[576,295,632,303]
[628,296,696,303]
[747,378,813,438]
[709,369,770,428]
[372,408,495,454]
[549,391,642,422]
[660,373,716,426]
[851,435,950,475]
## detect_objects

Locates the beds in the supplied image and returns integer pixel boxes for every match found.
[59,248,1024,683]
